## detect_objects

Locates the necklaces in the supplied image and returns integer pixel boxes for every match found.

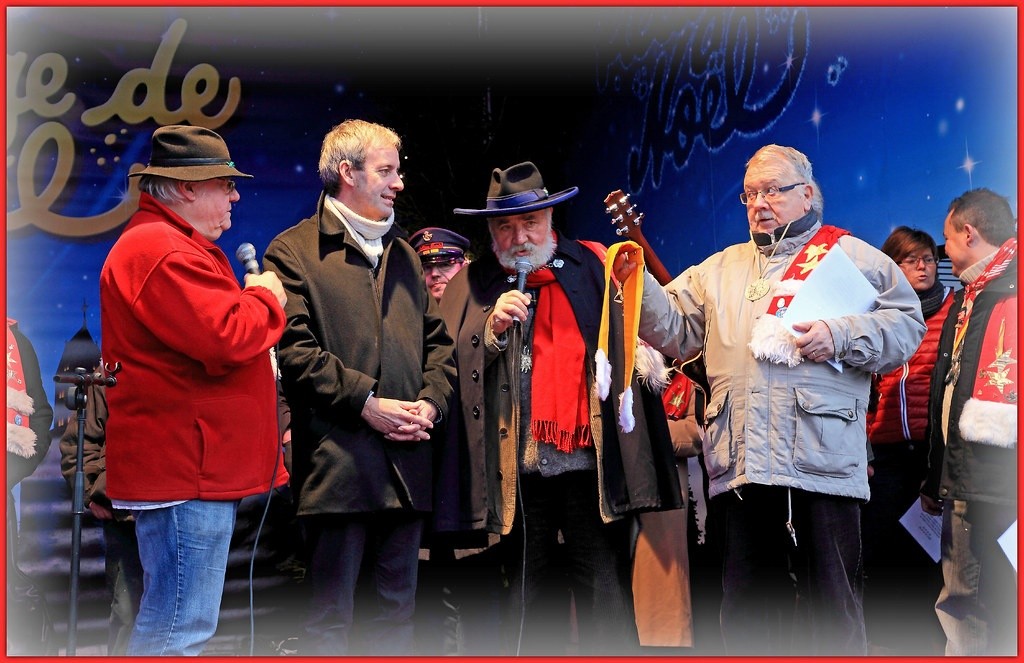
[745,277,770,300]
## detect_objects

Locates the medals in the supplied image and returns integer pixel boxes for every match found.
[944,363,954,382]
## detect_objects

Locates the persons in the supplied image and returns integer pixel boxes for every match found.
[100,124,289,656]
[852,186,1018,658]
[265,121,459,657]
[614,144,927,655]
[408,163,708,656]
[7,317,149,658]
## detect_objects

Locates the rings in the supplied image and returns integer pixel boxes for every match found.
[813,352,818,359]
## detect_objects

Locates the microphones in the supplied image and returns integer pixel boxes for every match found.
[512,257,533,327]
[236,242,261,276]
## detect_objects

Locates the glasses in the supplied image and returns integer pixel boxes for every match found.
[740,182,805,204]
[895,256,939,268]
[422,263,454,271]
[214,178,235,194]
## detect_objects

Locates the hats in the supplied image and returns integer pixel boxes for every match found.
[127,125,254,182]
[409,228,470,268]
[453,162,579,217]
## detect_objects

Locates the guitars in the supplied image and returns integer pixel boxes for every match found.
[605,187,673,286]
